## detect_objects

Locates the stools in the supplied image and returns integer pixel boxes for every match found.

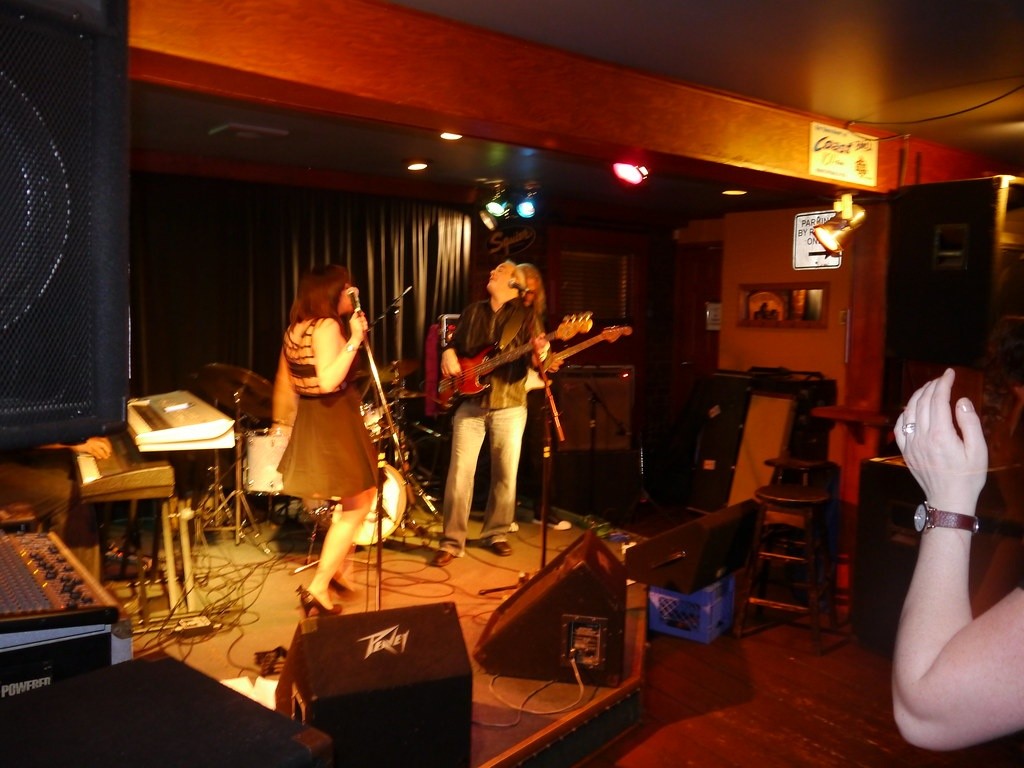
[734,453,827,643]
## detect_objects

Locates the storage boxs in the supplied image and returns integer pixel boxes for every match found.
[645,573,738,644]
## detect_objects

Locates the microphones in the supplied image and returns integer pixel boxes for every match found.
[615,430,633,437]
[508,280,530,292]
[347,288,361,314]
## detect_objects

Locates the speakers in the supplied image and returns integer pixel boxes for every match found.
[473,529,633,688]
[530,452,641,524]
[555,365,634,450]
[275,602,473,768]
[624,496,761,608]
[0,0,135,447]
[0,650,332,768]
[885,172,1024,373]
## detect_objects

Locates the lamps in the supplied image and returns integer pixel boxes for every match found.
[516,189,540,219]
[813,193,868,255]
[486,192,509,217]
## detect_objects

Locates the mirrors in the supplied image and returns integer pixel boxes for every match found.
[736,280,832,328]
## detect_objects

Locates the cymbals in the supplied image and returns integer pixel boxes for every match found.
[380,359,421,384]
[196,361,275,421]
[386,390,428,399]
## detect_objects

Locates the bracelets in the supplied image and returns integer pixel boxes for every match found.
[345,345,355,351]
[273,419,286,423]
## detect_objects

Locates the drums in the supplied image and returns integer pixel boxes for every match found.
[243,427,291,498]
[302,463,407,547]
[361,400,396,445]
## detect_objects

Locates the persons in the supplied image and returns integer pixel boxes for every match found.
[890,368,1024,752]
[0,437,154,571]
[506,264,572,531]
[270,264,380,618]
[428,262,551,566]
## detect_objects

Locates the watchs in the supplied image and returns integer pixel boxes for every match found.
[914,501,979,534]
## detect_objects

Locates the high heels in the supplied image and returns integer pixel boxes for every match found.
[327,577,358,599]
[301,590,341,618]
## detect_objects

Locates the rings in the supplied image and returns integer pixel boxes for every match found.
[903,423,916,435]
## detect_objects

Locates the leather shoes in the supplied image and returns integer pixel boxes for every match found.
[491,542,511,556]
[430,551,454,566]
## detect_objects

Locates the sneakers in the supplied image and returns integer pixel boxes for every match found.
[532,509,572,530]
[508,521,519,532]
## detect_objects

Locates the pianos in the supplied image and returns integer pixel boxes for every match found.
[68,388,238,635]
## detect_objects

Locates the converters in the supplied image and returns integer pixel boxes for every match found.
[178,617,215,639]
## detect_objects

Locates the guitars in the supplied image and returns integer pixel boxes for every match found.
[438,310,594,413]
[525,324,633,393]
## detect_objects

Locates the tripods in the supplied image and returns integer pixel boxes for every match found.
[195,391,272,556]
